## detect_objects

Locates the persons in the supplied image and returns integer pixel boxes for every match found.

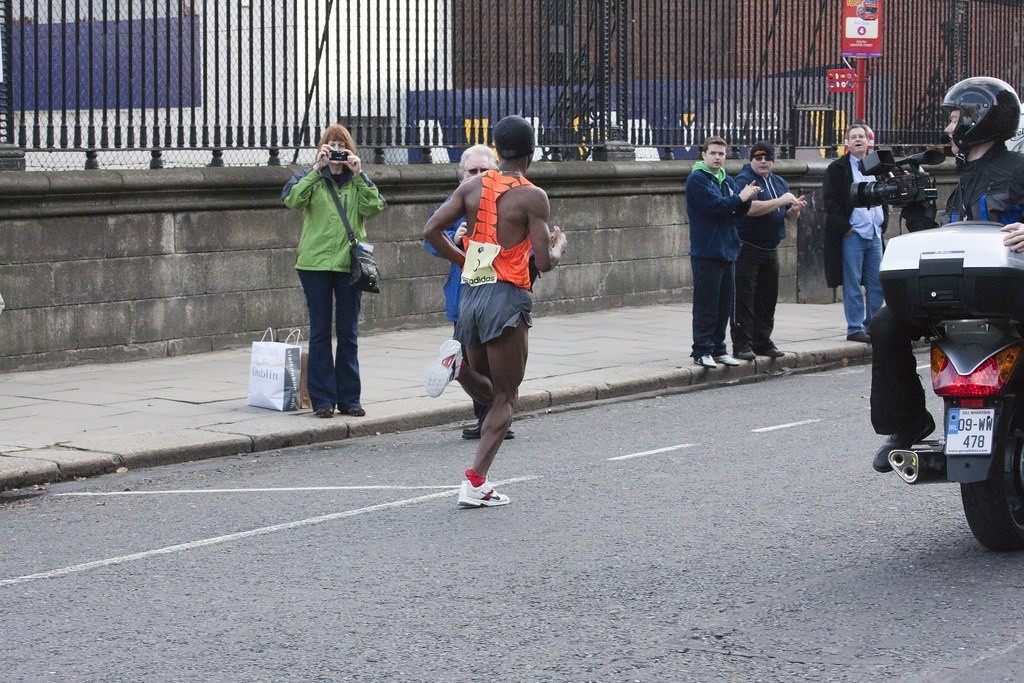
[869,77,1024,473]
[281,125,388,418]
[686,136,762,367]
[422,115,566,506]
[822,125,890,342]
[729,142,807,359]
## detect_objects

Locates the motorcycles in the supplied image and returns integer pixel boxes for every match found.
[876,220,1024,554]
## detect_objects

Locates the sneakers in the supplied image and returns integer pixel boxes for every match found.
[424,340,463,398]
[873,408,935,473]
[458,469,510,506]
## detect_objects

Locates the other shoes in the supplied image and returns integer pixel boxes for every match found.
[847,331,871,342]
[316,408,334,418]
[755,347,784,357]
[694,355,717,368]
[462,425,481,438]
[341,407,365,416]
[738,350,756,359]
[504,429,514,438]
[714,355,739,366]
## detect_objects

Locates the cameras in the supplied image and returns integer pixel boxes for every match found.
[329,151,347,160]
[850,149,946,221]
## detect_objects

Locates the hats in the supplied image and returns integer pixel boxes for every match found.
[750,141,774,162]
[495,115,535,160]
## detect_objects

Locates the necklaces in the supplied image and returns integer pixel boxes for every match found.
[496,166,521,174]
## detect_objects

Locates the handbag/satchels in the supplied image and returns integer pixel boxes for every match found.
[348,245,380,293]
[247,327,312,412]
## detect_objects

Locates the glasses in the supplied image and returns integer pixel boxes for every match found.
[464,168,489,174]
[755,156,770,161]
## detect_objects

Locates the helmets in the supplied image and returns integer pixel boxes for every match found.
[940,77,1021,149]
